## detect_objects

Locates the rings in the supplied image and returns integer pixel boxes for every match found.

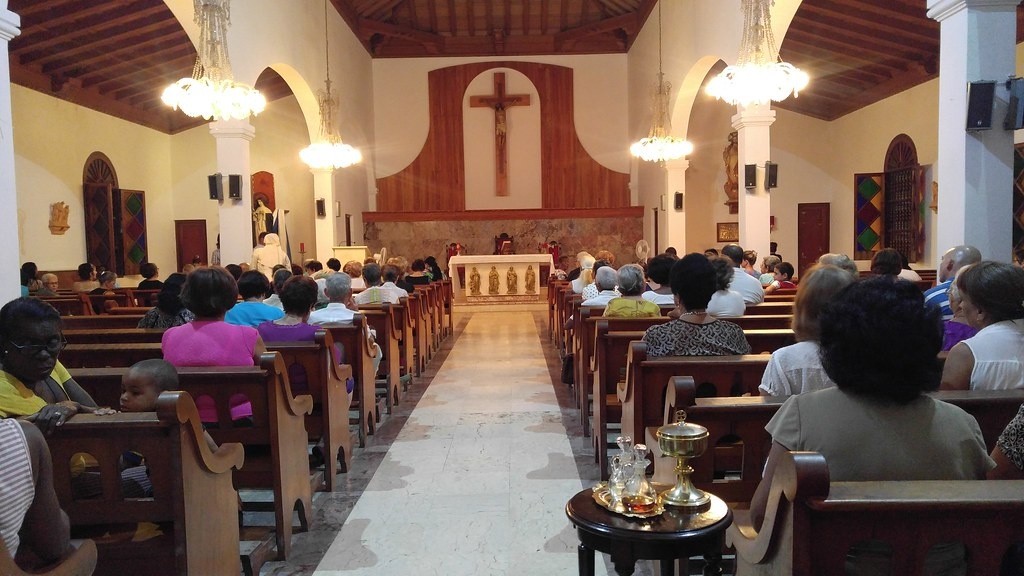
[54,411,62,415]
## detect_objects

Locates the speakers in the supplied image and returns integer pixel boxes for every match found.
[674,193,683,209]
[764,163,777,189]
[966,81,995,130]
[745,164,756,189]
[209,174,223,200]
[1004,78,1024,130]
[229,175,242,199]
[316,200,325,216]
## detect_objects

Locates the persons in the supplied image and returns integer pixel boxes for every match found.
[723,131,738,190]
[489,266,499,294]
[932,182,938,205]
[507,266,517,291]
[137,233,448,470]
[73,263,117,315]
[253,193,272,246]
[525,265,535,291]
[0,296,243,562]
[565,241,797,360]
[21,262,60,296]
[470,267,480,294]
[750,245,1024,534]
[51,201,69,225]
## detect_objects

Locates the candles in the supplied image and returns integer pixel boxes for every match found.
[300,242,305,252]
[456,241,461,252]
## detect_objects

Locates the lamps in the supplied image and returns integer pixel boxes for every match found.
[161,0,266,124]
[631,0,695,162]
[706,0,810,105]
[299,0,364,168]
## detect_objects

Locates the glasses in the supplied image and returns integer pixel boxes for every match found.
[7,331,68,355]
[46,282,59,285]
[945,288,953,296]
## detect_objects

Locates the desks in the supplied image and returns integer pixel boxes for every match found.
[564,486,733,576]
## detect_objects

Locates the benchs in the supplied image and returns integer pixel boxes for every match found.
[546,243,1024,575]
[0,272,455,576]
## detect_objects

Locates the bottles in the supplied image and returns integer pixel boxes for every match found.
[609,436,656,513]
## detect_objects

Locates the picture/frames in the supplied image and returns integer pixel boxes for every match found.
[717,222,738,243]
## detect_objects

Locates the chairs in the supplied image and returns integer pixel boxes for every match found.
[443,242,466,286]
[494,233,515,254]
[537,240,570,282]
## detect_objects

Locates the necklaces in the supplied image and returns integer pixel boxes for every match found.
[679,311,708,319]
[27,379,57,403]
